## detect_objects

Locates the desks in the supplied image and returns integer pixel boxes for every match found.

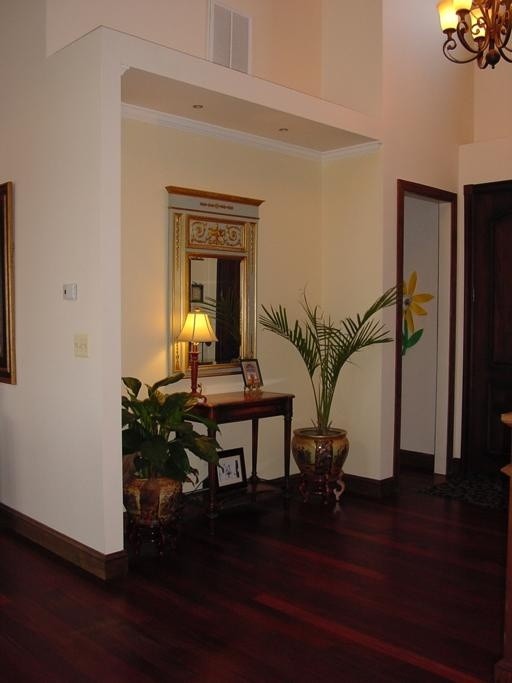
[184,389,295,536]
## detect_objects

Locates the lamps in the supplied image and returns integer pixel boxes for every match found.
[176,307,219,398]
[437,0,511,70]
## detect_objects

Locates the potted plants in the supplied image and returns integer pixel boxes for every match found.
[257,281,399,514]
[121,372,226,560]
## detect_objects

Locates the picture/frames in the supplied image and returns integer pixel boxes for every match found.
[239,359,264,393]
[192,285,203,303]
[0,181,17,386]
[216,447,248,493]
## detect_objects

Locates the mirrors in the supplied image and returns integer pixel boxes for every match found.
[165,185,265,379]
